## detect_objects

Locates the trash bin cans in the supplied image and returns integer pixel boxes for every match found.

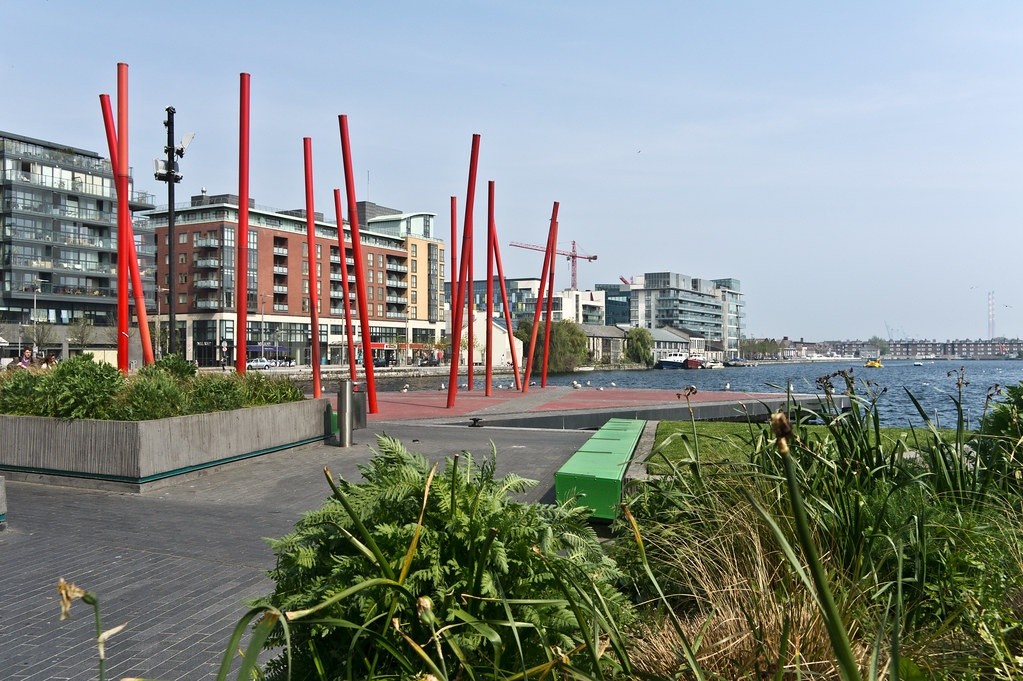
[337,381,366,430]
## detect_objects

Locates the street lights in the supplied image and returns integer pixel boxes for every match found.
[30,284,40,346]
[261,292,274,358]
[157,286,170,356]
[406,305,410,365]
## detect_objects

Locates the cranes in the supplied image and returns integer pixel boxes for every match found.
[508,239,598,290]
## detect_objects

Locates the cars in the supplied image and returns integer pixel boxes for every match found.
[281,360,296,367]
[421,357,439,367]
[362,358,386,368]
[246,359,270,371]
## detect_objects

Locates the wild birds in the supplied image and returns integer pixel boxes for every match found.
[401,378,732,393]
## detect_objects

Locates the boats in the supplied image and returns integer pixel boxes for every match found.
[863,358,884,368]
[914,362,923,366]
[657,352,705,370]
[727,361,759,366]
[683,357,706,369]
[706,361,724,369]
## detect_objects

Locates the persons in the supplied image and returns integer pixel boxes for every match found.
[7,347,41,370]
[41,353,58,370]
[220,356,227,371]
